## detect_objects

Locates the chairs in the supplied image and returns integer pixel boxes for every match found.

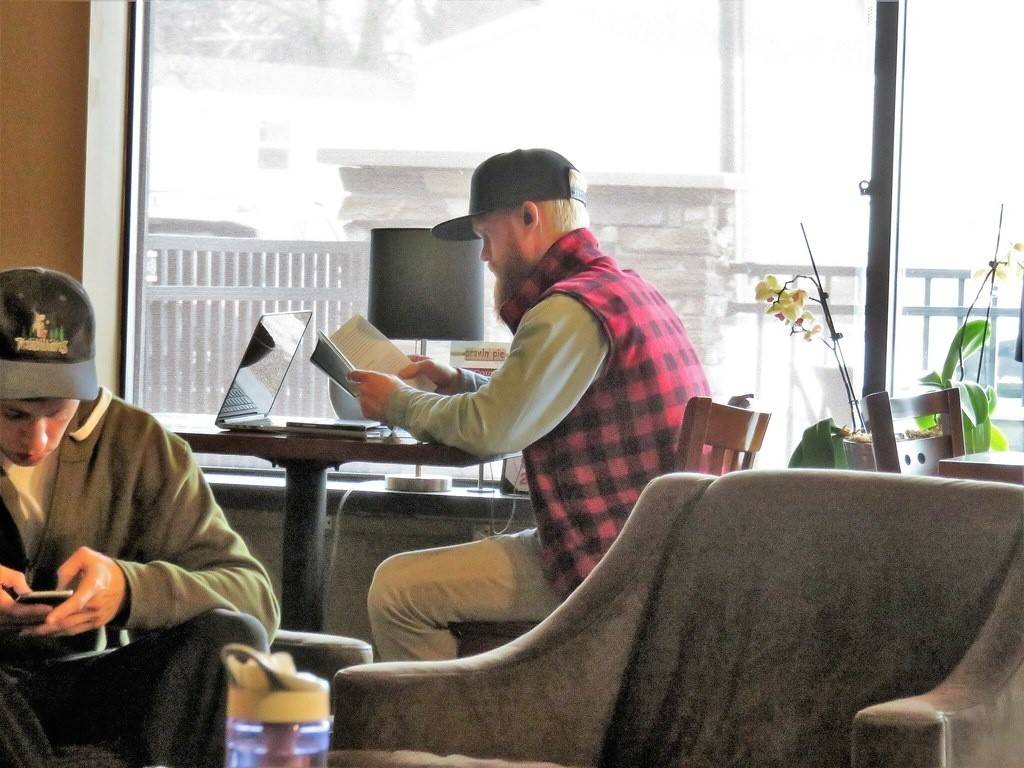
[447,393,772,659]
[859,388,965,474]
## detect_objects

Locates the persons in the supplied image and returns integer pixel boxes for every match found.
[346,149,726,662]
[0,267,281,768]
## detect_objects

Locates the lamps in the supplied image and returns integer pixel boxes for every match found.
[368,229,483,491]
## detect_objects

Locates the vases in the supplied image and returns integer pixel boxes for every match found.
[842,434,904,472]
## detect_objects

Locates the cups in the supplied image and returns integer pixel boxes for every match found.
[226,677,330,767]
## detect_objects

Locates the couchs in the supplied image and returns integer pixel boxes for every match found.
[55,631,372,768]
[327,469,1024,768]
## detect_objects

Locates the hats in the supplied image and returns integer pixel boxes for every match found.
[0,266,99,401]
[431,149,588,241]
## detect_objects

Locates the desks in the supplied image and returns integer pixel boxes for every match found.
[170,427,523,634]
[938,451,1024,485]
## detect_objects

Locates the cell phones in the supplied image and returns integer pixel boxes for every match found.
[16,591,73,605]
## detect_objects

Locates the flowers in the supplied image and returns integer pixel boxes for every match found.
[753,222,874,442]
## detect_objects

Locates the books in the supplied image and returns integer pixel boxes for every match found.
[309,313,438,432]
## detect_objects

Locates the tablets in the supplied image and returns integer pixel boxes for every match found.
[214,310,381,439]
[286,420,381,431]
